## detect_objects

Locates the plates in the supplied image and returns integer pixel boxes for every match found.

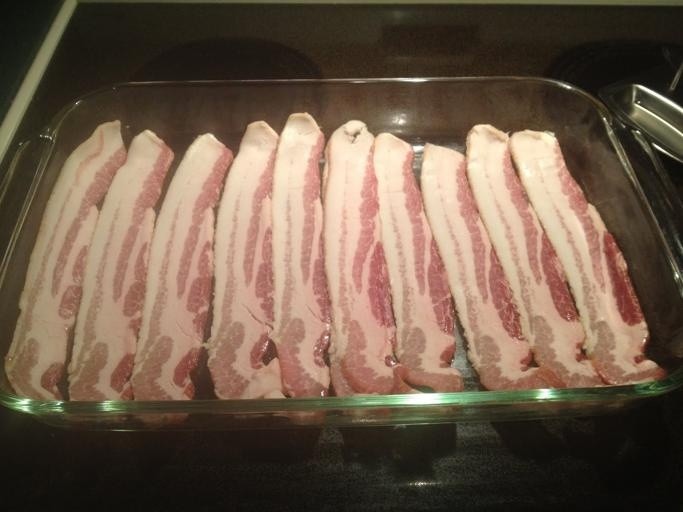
[0,77,682,429]
